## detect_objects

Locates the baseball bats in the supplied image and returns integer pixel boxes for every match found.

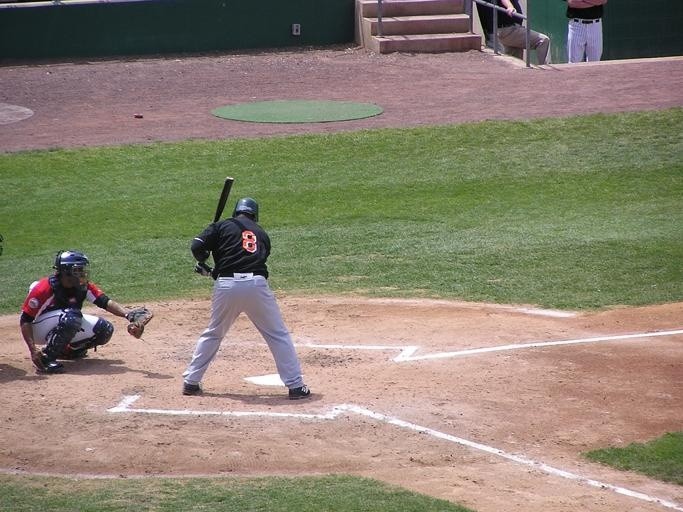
[196,177,234,273]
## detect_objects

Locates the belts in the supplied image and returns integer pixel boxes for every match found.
[219,271,266,277]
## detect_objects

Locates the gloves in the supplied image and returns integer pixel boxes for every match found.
[193,261,215,276]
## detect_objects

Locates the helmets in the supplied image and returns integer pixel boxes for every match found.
[232,197,258,222]
[52,250,89,270]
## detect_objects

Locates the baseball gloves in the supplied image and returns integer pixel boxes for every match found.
[127,306,154,340]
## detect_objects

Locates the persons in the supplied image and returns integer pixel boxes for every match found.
[181,198,311,400]
[20,251,131,371]
[486,0,552,65]
[566,0,607,63]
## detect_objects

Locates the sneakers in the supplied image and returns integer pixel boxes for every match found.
[183,383,203,396]
[35,353,63,373]
[289,386,311,400]
